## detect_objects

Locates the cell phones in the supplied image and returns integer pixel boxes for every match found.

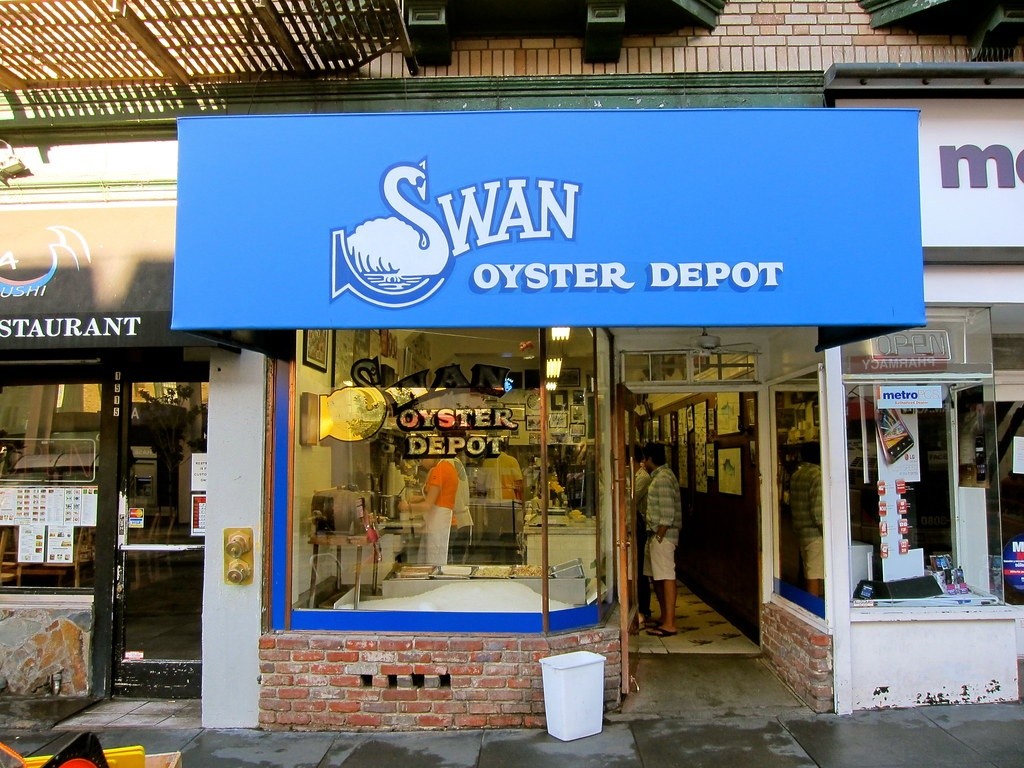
[975,436,986,481]
[859,584,874,599]
[929,554,965,585]
[876,409,914,464]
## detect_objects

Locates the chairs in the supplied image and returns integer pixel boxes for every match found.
[0,507,177,590]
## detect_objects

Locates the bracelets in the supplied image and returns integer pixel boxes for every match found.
[656,535,662,543]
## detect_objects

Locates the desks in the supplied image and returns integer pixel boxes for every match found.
[468,497,521,546]
[379,514,425,563]
[305,525,387,609]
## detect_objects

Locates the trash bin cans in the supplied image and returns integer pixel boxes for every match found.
[538,651,607,742]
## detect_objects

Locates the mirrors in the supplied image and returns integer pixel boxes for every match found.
[763,362,833,635]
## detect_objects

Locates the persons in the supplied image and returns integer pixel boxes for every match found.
[398,457,474,564]
[625,442,682,636]
[477,440,525,565]
[790,441,824,597]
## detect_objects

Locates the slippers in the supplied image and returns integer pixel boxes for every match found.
[645,620,663,628]
[646,627,677,636]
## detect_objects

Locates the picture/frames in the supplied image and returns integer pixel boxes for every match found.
[302,327,430,396]
[774,390,818,446]
[641,391,746,497]
[504,364,585,444]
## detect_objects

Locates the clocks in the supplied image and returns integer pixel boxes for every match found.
[527,393,541,409]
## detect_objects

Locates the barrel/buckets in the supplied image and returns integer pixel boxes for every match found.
[539,650,607,741]
[380,494,398,518]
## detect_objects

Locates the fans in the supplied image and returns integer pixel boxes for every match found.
[673,328,762,358]
